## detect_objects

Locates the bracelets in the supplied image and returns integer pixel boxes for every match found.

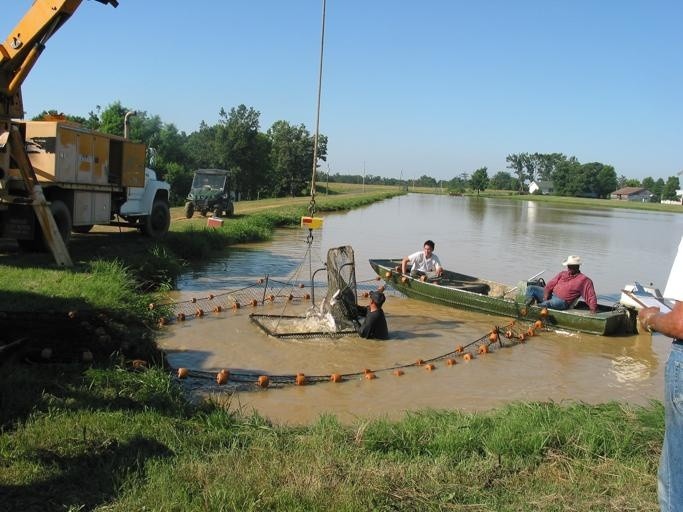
[644,313,655,335]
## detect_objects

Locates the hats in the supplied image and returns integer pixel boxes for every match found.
[369,291,385,304]
[563,256,582,265]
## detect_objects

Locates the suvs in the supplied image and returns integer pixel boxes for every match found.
[183,168,234,217]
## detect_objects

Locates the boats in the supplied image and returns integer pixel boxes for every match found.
[368,257,662,338]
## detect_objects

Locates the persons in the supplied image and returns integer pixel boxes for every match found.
[348,289,389,339]
[401,240,443,285]
[637,235,683,511]
[525,254,597,312]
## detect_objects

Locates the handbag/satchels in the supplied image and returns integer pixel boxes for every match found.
[527,278,545,287]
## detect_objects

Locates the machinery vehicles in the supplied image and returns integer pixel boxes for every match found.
[0,0,171,252]
